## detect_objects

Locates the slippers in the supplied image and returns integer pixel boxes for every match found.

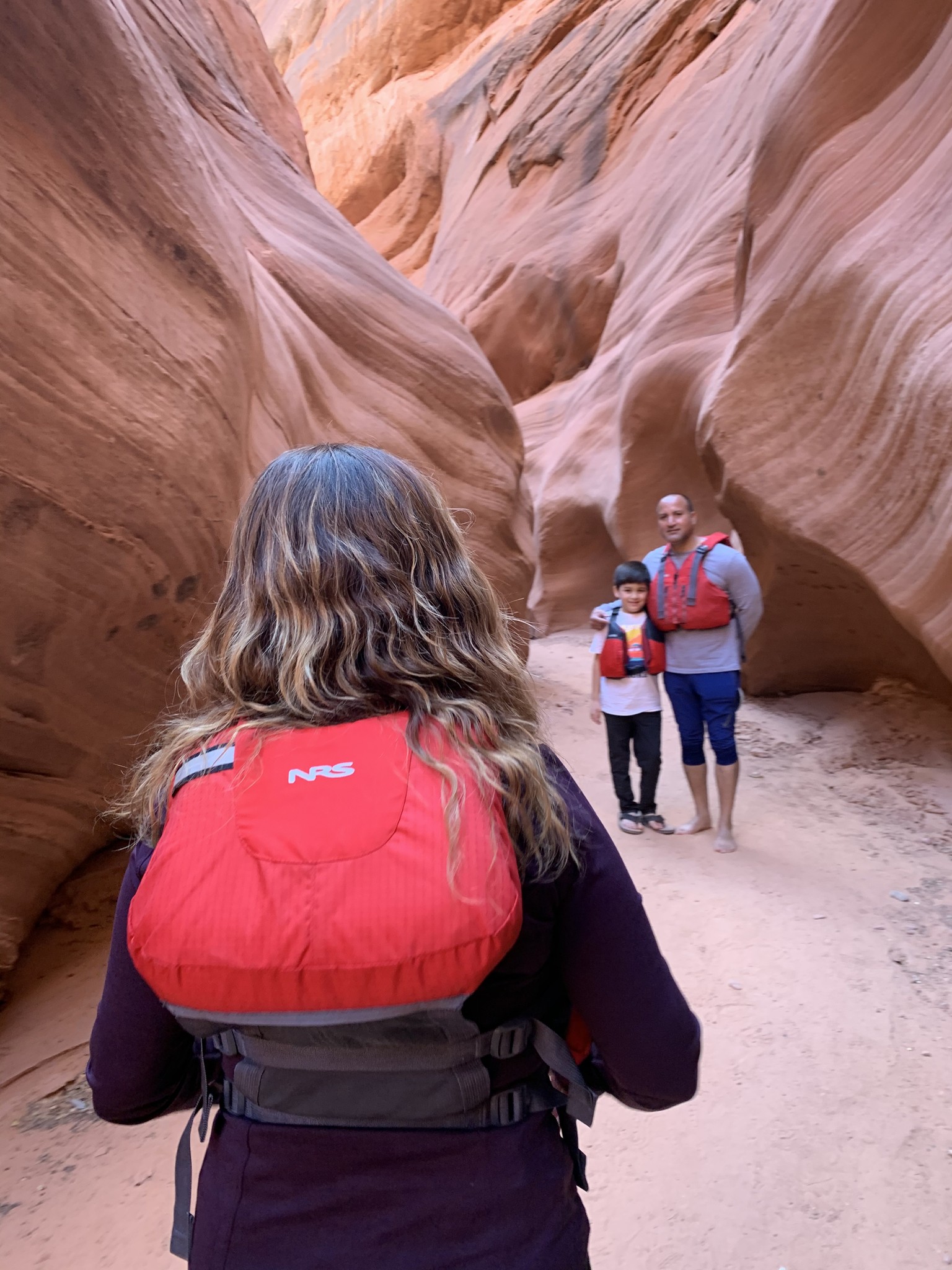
[617,811,644,834]
[643,811,674,833]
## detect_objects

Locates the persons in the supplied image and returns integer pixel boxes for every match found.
[590,559,677,834]
[591,493,763,854]
[82,442,704,1270]
